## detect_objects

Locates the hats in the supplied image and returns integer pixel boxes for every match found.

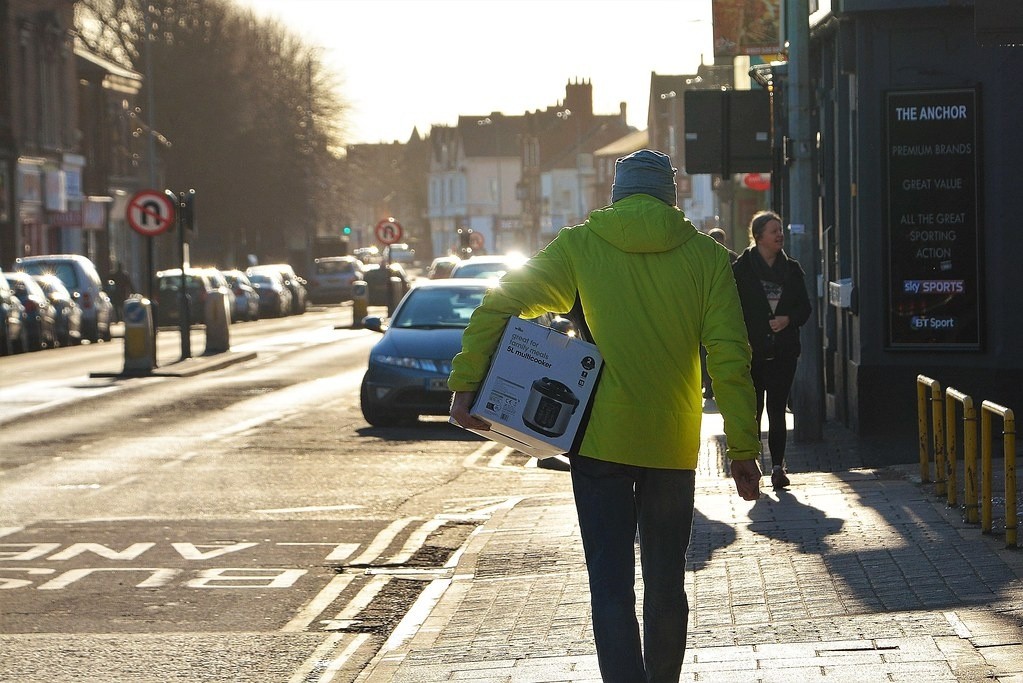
[611,149,677,205]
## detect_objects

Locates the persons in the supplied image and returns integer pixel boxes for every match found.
[702,228,738,400]
[109,262,135,324]
[449,150,763,683]
[730,212,813,489]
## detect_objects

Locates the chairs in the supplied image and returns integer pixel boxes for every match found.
[413,305,441,325]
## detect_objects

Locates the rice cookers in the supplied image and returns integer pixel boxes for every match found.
[522,376,580,438]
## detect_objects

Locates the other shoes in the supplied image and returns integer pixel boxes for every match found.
[771,465,790,490]
[703,388,714,397]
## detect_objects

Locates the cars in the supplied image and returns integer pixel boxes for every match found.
[4,271,56,352]
[447,254,532,320]
[358,249,384,265]
[358,263,411,318]
[153,268,236,326]
[245,264,307,316]
[32,275,83,349]
[0,269,28,355]
[359,277,572,426]
[382,244,416,269]
[306,256,364,304]
[426,257,462,279]
[221,270,260,324]
[12,254,115,343]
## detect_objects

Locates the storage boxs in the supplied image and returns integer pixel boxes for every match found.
[447,316,600,460]
